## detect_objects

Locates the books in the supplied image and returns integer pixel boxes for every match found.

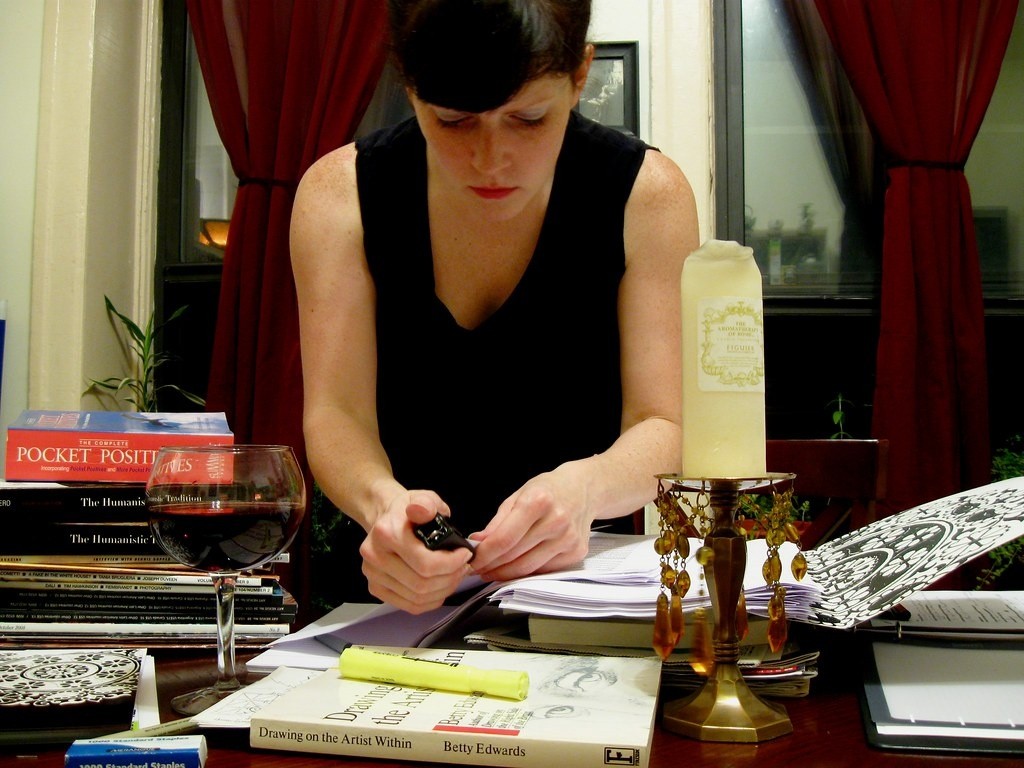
[485,643,819,699]
[0,410,297,649]
[852,588,1023,758]
[250,645,663,768]
[0,649,161,749]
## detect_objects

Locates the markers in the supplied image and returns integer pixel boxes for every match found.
[314,631,404,658]
[340,646,532,701]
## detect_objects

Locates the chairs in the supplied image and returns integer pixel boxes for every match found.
[634,439,879,551]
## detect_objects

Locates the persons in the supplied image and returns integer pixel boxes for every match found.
[290,0,701,634]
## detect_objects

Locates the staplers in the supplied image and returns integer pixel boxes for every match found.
[412,512,476,566]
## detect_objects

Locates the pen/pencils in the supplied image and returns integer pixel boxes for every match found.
[879,603,912,622]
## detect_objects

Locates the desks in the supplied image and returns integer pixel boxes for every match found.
[1,645,1024,768]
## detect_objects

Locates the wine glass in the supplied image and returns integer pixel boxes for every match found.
[145,444,306,717]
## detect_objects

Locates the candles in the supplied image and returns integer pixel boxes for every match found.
[680,239,767,479]
